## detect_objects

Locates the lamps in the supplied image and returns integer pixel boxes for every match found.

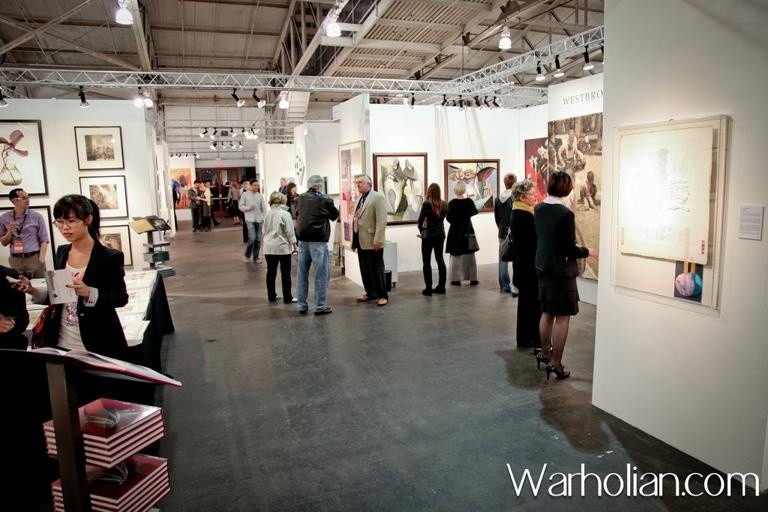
[499,23,513,50]
[230,87,289,109]
[0,90,8,108]
[533,43,603,82]
[114,0,133,26]
[170,127,256,159]
[326,15,342,37]
[78,86,90,109]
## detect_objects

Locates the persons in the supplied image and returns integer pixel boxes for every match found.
[445,181,480,287]
[493,172,517,297]
[0,186,51,280]
[527,125,602,213]
[173,178,299,265]
[263,190,298,304]
[351,174,389,306]
[533,171,601,381]
[0,264,30,350]
[417,183,450,297]
[507,180,538,356]
[295,174,340,316]
[9,192,132,422]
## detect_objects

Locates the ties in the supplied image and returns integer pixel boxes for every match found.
[353,197,364,233]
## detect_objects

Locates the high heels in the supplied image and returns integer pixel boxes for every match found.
[536,352,570,380]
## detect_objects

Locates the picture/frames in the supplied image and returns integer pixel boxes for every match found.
[75,126,125,169]
[0,118,47,195]
[97,225,132,265]
[0,206,54,278]
[80,175,128,218]
[337,138,367,248]
[444,158,500,211]
[372,153,429,227]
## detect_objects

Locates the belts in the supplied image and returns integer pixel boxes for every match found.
[11,250,39,259]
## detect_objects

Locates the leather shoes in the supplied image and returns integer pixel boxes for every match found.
[378,299,388,305]
[357,295,371,301]
[534,349,542,356]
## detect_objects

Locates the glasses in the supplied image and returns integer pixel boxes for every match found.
[14,196,29,200]
[52,219,81,228]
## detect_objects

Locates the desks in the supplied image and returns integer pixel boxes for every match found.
[25,271,176,406]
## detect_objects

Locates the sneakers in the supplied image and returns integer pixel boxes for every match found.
[193,222,221,233]
[292,251,298,255]
[422,280,478,296]
[270,296,332,315]
[243,255,263,264]
[500,290,510,293]
[234,222,240,225]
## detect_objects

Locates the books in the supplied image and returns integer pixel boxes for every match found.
[42,396,172,512]
[143,250,176,278]
[24,345,183,389]
[128,218,172,235]
[142,241,170,248]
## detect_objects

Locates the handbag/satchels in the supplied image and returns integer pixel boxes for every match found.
[422,217,428,229]
[500,233,514,262]
[31,304,55,349]
[193,208,201,214]
[465,233,479,251]
[225,198,233,211]
